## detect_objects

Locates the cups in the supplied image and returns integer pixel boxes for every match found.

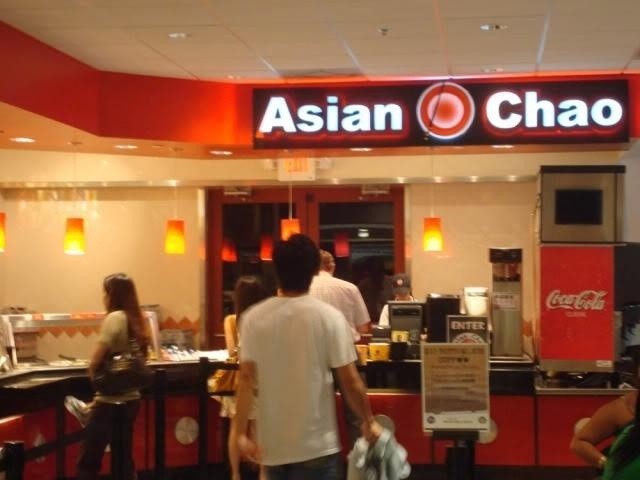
[355,343,391,365]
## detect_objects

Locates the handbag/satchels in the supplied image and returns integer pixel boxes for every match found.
[207,345,257,420]
[90,349,154,397]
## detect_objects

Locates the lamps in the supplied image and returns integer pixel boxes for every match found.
[163,156,187,255]
[63,150,88,255]
[422,150,444,250]
[278,157,301,241]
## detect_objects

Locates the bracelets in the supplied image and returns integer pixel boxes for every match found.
[598,455,609,472]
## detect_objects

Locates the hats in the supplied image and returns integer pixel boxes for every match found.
[390,272,413,296]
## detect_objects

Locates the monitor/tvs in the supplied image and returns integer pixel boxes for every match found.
[387,300,429,333]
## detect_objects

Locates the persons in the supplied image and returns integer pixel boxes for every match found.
[354,253,393,322]
[69,272,152,480]
[228,231,383,480]
[601,389,640,479]
[304,247,371,455]
[568,345,640,472]
[379,272,421,330]
[218,273,271,480]
